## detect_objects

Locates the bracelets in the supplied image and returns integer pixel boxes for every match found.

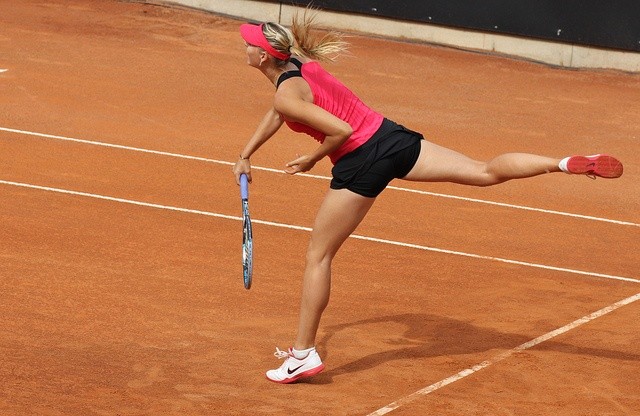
[239,154,250,160]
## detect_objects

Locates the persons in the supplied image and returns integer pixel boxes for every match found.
[232,4,624,383]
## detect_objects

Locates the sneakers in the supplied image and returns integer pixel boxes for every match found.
[558,154,623,180]
[265,346,325,384]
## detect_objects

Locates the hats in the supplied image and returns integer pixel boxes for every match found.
[239,23,289,61]
